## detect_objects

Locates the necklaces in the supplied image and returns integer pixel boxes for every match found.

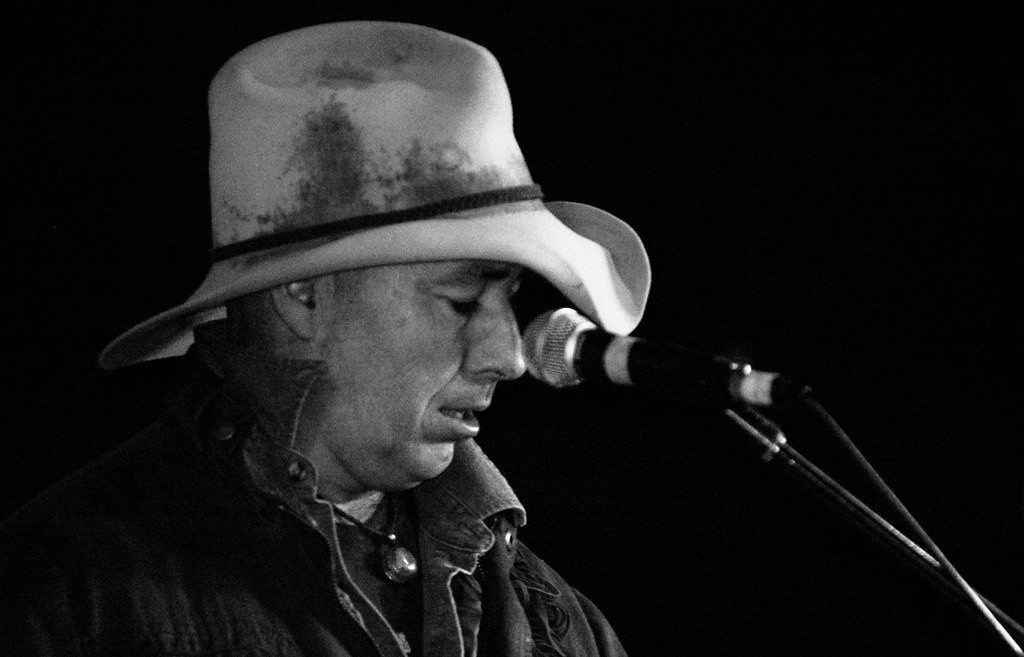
[325,495,418,586]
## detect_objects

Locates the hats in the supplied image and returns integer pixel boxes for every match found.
[103,20,651,400]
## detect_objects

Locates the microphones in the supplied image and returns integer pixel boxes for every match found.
[522,311,814,411]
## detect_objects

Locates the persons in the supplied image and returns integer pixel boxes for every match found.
[0,20,651,657]
